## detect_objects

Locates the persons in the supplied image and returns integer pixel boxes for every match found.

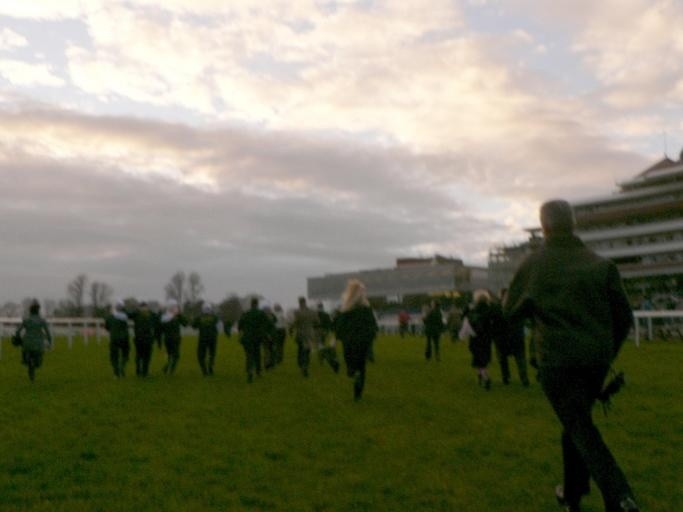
[422,297,464,362]
[490,290,539,387]
[221,317,234,337]
[288,296,340,377]
[190,303,219,377]
[238,297,289,383]
[499,200,644,510]
[121,299,188,376]
[326,279,379,402]
[15,297,52,381]
[460,289,494,389]
[105,302,131,378]
[398,307,415,339]
